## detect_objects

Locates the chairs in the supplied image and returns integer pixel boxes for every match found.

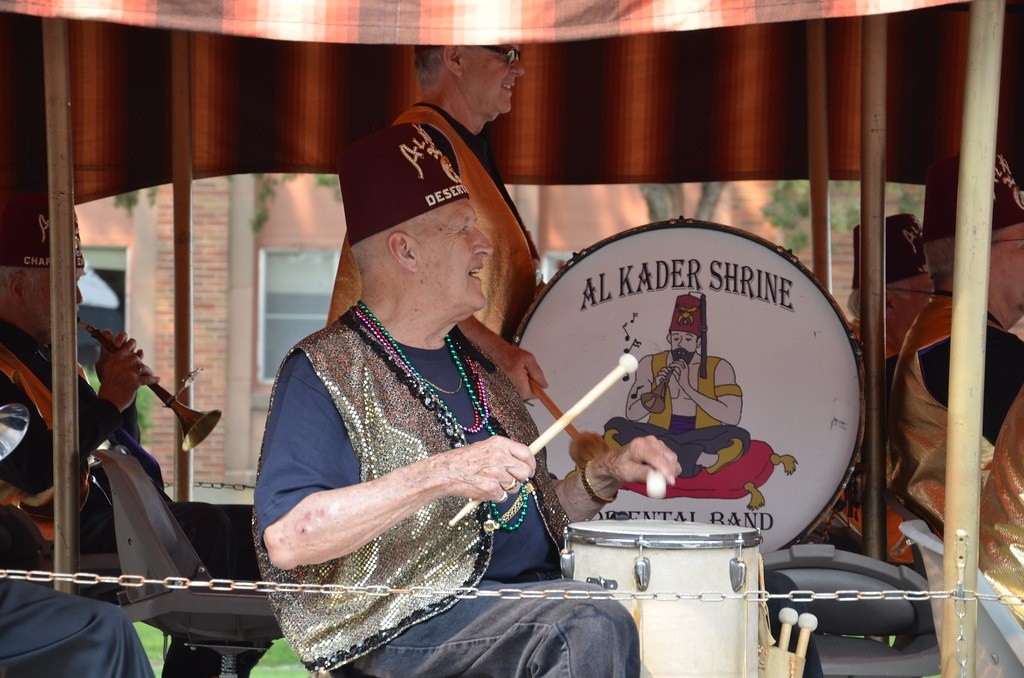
[0,448,287,678]
[761,486,1024,678]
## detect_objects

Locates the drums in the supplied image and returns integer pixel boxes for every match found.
[508,215,867,556]
[559,521,762,678]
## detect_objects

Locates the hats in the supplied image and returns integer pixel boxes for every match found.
[336,121,471,246]
[849,213,925,289]
[1,187,86,270]
[920,150,1024,244]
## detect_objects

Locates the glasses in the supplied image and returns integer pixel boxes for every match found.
[481,45,521,66]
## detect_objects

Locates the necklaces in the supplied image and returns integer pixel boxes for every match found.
[354,301,528,531]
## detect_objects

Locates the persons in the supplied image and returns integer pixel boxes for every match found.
[251,120,682,678]
[0,189,271,678]
[325,44,548,400]
[831,153,1024,633]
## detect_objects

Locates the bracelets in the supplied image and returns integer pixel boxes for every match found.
[581,460,618,503]
